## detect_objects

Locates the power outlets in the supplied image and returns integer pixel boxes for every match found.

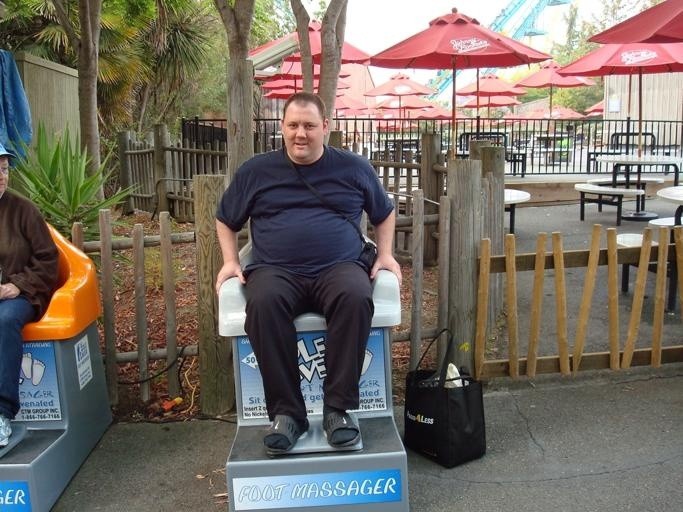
[162,397,184,414]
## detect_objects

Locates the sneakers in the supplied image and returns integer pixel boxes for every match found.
[1,415,11,446]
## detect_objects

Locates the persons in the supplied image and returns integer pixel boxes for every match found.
[1,140,60,449]
[215,92,402,454]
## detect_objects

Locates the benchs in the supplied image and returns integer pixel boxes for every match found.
[527,148,575,167]
[574,183,646,227]
[617,233,678,310]
[592,178,665,212]
[387,187,531,233]
[442,151,532,177]
[589,148,678,175]
[648,217,682,244]
[376,138,421,151]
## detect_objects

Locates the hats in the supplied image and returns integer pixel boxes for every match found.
[0,144,11,157]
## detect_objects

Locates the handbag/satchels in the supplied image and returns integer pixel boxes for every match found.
[283,145,376,270]
[405,329,486,469]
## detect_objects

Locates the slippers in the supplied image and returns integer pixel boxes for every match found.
[322,411,360,447]
[263,415,306,454]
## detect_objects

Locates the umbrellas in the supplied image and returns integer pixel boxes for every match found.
[437,113,470,124]
[457,96,522,109]
[414,109,456,120]
[371,7,554,160]
[364,72,434,121]
[556,44,683,158]
[261,80,368,117]
[584,100,604,111]
[514,62,598,136]
[368,95,435,110]
[247,19,372,91]
[588,1,683,45]
[543,106,587,119]
[455,74,528,121]
[255,62,350,94]
[588,112,605,116]
